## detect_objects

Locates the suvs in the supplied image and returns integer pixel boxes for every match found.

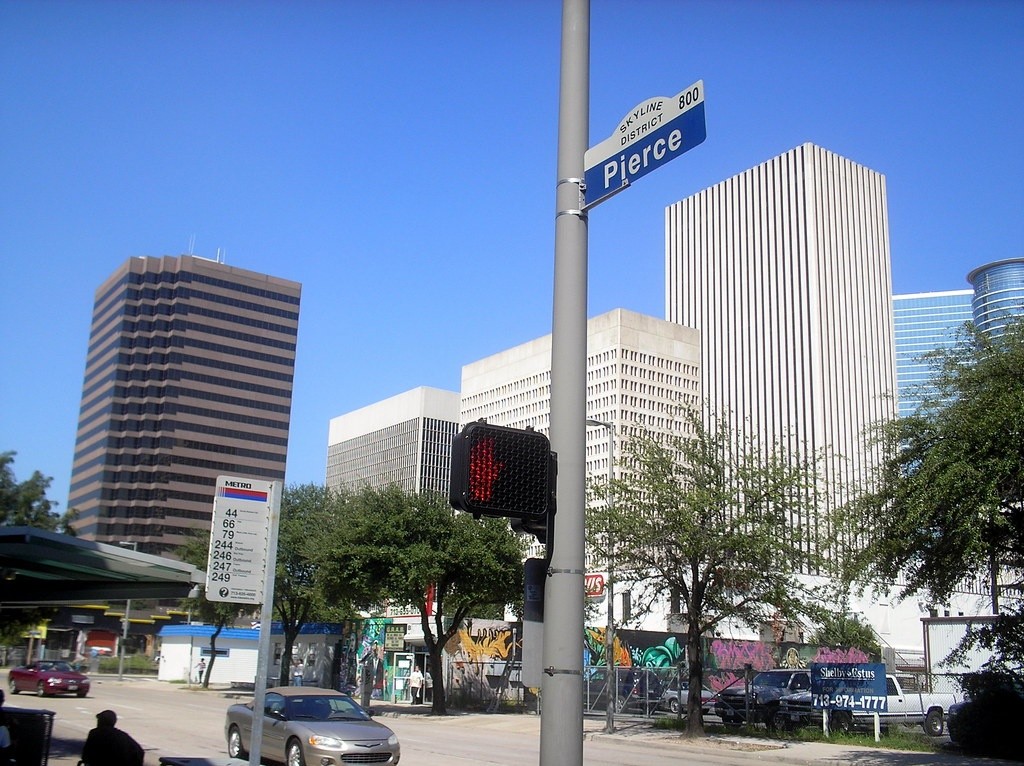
[714,668,812,732]
[583,667,665,714]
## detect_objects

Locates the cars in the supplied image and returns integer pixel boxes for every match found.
[224,686,401,766]
[8,658,91,698]
[664,682,718,714]
[947,684,1024,744]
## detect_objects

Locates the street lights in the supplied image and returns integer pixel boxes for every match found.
[117,540,138,681]
[584,419,617,734]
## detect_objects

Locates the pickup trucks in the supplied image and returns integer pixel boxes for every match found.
[777,673,956,737]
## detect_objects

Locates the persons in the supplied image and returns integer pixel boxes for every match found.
[291,658,305,688]
[0,690,11,766]
[409,666,422,705]
[195,658,205,683]
[82,710,145,766]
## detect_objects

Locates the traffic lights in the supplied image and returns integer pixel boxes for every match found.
[447,420,550,523]
